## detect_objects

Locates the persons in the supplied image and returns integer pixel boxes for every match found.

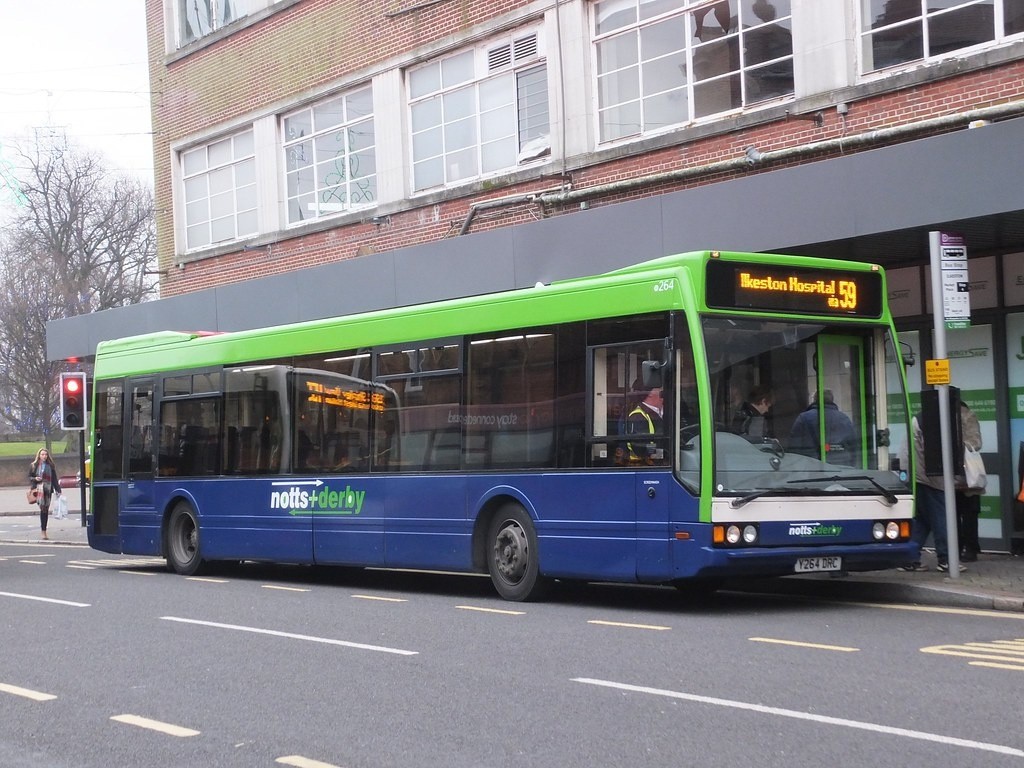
[28,450,61,540]
[732,386,775,448]
[896,397,983,573]
[789,389,857,467]
[625,384,669,467]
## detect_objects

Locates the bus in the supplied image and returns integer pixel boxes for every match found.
[79,250,914,601]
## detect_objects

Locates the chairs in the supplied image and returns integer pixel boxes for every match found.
[107,424,312,470]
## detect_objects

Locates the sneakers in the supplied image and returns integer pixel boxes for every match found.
[935,561,967,573]
[896,560,932,572]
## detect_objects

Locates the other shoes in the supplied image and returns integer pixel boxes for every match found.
[41,536,49,541]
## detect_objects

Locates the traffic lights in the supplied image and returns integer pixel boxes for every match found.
[59,372,86,430]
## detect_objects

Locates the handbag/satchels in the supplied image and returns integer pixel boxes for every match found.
[963,443,988,489]
[27,476,40,510]
[47,487,69,520]
